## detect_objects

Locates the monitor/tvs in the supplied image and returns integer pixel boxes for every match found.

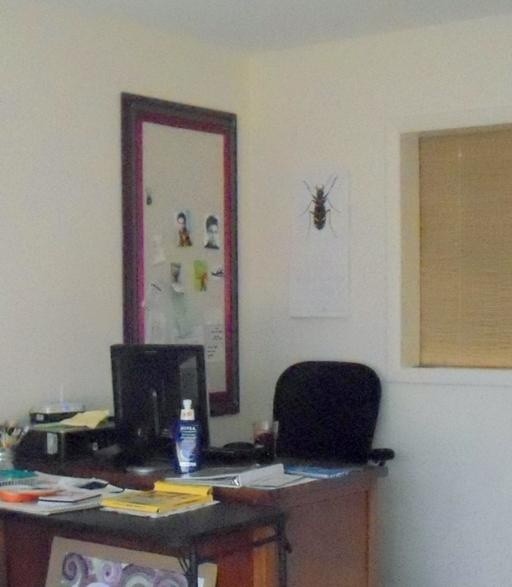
[101,481,214,516]
[287,464,349,481]
[0,464,148,516]
[166,464,284,490]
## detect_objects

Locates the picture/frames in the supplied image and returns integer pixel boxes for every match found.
[0,459,389,587]
[0,468,290,587]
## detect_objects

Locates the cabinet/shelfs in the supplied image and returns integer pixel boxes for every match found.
[173,397,203,474]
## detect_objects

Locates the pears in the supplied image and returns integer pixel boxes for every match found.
[175,399,203,473]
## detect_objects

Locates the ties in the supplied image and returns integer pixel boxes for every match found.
[110,344,209,474]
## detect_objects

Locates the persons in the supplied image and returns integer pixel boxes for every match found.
[195,272,207,291]
[177,212,190,247]
[170,264,181,285]
[204,216,219,249]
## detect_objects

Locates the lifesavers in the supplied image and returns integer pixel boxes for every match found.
[8,418,116,461]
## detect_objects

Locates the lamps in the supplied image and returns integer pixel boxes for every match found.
[219,359,398,469]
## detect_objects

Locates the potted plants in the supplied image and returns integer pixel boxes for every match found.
[118,88,244,419]
[42,532,221,587]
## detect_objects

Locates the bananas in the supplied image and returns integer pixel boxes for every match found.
[165,446,253,461]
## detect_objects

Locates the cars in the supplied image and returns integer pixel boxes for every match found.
[250,419,282,463]
